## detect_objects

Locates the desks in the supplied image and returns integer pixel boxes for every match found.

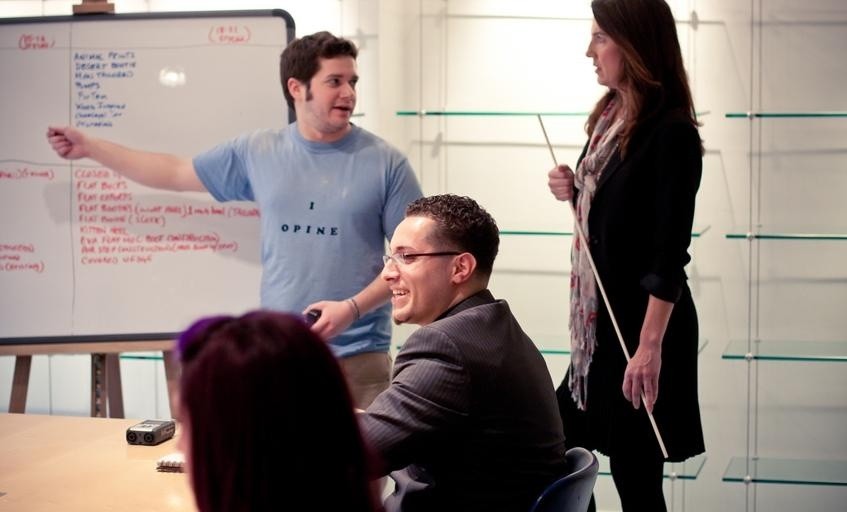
[0,410,200,512]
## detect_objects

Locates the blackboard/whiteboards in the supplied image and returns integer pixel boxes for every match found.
[0,9,295,346]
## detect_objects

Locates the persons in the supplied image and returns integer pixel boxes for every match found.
[351,191,567,512]
[173,307,388,512]
[44,28,423,412]
[547,0,708,511]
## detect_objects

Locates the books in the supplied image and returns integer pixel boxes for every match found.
[156,449,188,474]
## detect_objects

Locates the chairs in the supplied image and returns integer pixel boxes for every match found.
[530,446,600,512]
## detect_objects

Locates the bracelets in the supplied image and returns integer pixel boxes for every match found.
[343,299,358,323]
[351,297,360,323]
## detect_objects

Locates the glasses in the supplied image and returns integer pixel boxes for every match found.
[380,248,462,268]
